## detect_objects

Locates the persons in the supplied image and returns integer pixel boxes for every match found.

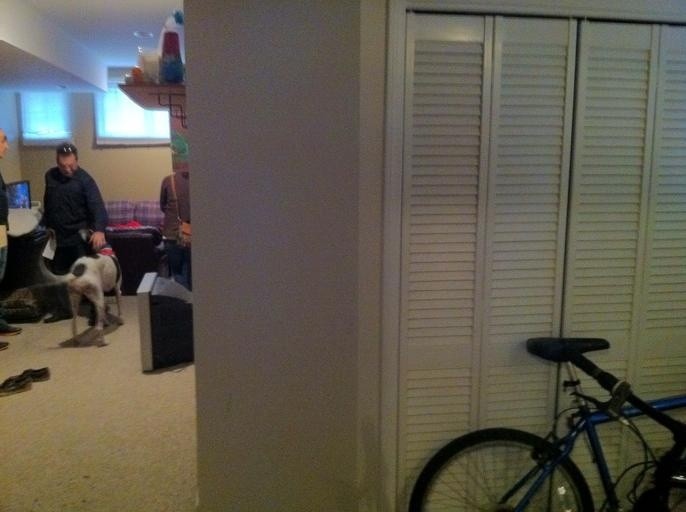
[159,168,191,290]
[36,143,110,326]
[0,128,22,351]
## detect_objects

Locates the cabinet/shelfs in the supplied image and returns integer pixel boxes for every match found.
[560,4,685,511]
[381,3,581,512]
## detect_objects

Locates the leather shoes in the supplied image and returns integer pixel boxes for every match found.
[1,326,23,336]
[0,342,9,351]
[0,374,33,397]
[44,311,73,324]
[10,367,50,382]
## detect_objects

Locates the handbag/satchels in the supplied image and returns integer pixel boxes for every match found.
[179,221,191,249]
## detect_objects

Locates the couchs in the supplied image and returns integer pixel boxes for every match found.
[105,201,171,296]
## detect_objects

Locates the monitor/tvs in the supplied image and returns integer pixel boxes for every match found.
[137,272,194,372]
[6,180,31,209]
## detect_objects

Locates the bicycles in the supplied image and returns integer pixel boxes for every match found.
[410,330,686,511]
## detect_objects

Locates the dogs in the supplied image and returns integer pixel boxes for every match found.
[36,226,125,347]
